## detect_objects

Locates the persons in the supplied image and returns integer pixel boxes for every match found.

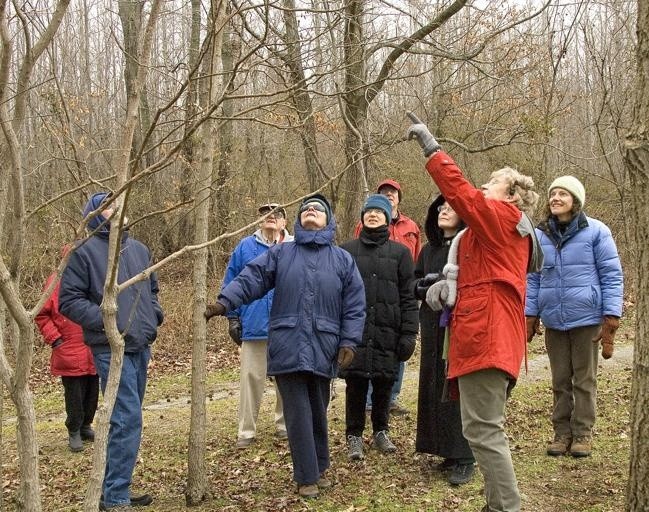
[57,190,166,512]
[193,192,368,500]
[33,242,103,453]
[220,203,293,449]
[407,192,477,485]
[354,177,422,418]
[404,109,545,511]
[337,193,420,460]
[523,175,625,457]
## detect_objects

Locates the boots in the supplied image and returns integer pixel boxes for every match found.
[81,427,95,441]
[69,428,83,452]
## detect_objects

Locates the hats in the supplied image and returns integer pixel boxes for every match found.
[377,179,401,194]
[93,192,113,225]
[547,175,585,211]
[299,198,330,224]
[361,194,391,226]
[259,203,288,219]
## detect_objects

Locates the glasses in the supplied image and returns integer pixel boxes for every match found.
[437,204,454,213]
[300,204,326,213]
[365,208,385,214]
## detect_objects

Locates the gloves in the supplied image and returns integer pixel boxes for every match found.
[337,348,355,370]
[406,110,441,158]
[204,302,225,324]
[426,280,448,311]
[395,334,417,362]
[526,317,542,342]
[416,272,440,299]
[227,317,242,347]
[592,316,619,359]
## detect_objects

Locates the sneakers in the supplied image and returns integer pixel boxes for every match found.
[235,399,475,498]
[570,437,593,456]
[546,432,572,455]
[99,494,153,512]
